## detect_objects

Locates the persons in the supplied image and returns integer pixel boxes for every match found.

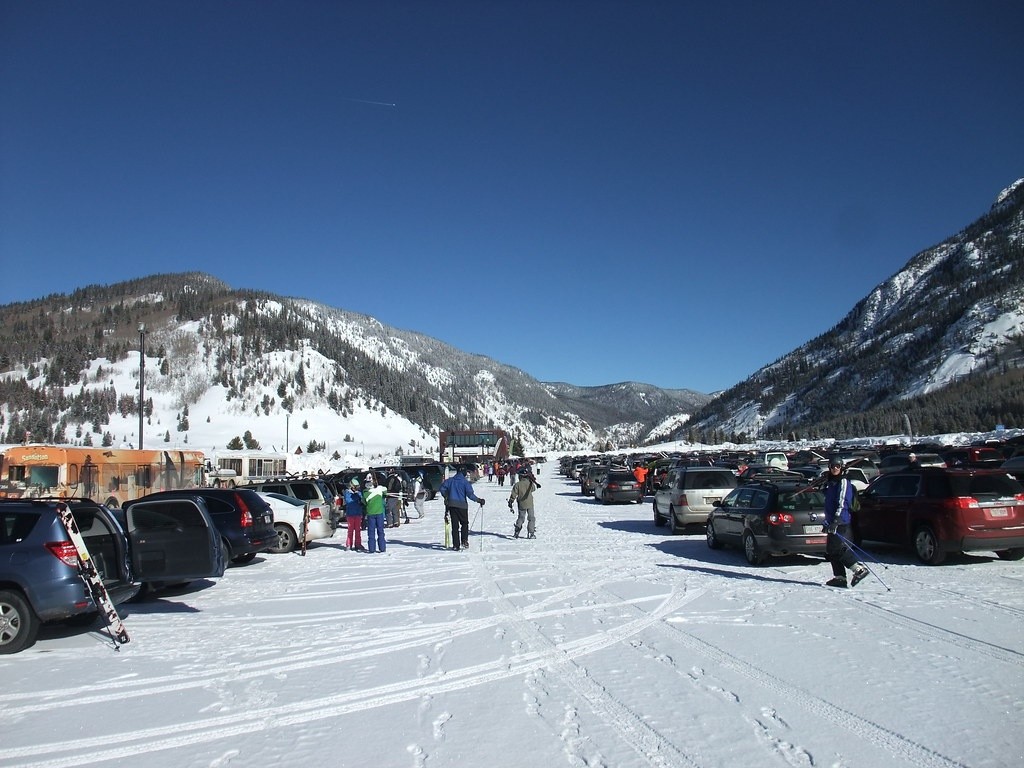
[439,465,485,550]
[484,458,533,486]
[908,453,921,468]
[634,464,648,496]
[821,455,870,588]
[508,469,536,539]
[303,468,427,553]
[657,469,667,487]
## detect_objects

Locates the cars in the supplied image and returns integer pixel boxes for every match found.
[705,473,855,568]
[847,467,1024,567]
[207,454,485,534]
[0,493,224,655]
[122,487,281,572]
[557,428,1024,506]
[253,488,334,554]
[652,464,739,535]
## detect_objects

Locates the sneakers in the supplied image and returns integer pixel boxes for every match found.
[355,545,364,551]
[346,546,352,550]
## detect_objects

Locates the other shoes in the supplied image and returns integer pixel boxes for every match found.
[528,532,536,539]
[462,541,469,548]
[826,578,847,588]
[453,545,460,551]
[385,524,393,528]
[393,523,399,527]
[851,568,870,587]
[418,514,424,519]
[514,528,520,539]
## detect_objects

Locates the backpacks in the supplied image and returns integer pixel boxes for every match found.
[824,475,861,513]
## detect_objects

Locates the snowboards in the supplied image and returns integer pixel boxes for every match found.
[55,503,131,645]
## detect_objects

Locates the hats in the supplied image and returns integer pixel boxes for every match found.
[349,479,360,487]
[829,456,843,464]
[516,470,528,476]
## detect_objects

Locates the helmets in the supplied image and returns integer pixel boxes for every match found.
[366,474,373,481]
[456,465,467,476]
[415,472,423,481]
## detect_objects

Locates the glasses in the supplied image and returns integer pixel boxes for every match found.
[830,464,840,469]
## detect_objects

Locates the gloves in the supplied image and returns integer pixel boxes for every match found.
[477,498,485,507]
[508,499,514,508]
[821,516,841,535]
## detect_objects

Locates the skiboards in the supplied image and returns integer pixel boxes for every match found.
[302,504,309,556]
[788,442,887,499]
[527,472,541,489]
[444,466,450,549]
[369,468,379,487]
